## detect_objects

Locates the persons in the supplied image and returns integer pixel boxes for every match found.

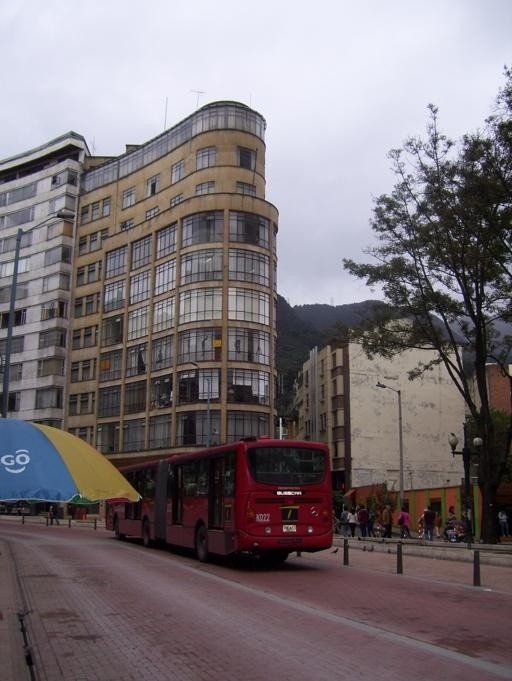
[332,503,472,543]
[498,508,511,538]
[50,503,59,525]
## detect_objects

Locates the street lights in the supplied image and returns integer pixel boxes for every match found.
[189,359,213,448]
[444,429,485,546]
[0,206,76,414]
[374,380,404,499]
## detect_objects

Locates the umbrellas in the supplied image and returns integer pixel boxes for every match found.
[0,418,142,506]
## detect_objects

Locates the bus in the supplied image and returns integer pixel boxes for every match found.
[100,431,337,567]
